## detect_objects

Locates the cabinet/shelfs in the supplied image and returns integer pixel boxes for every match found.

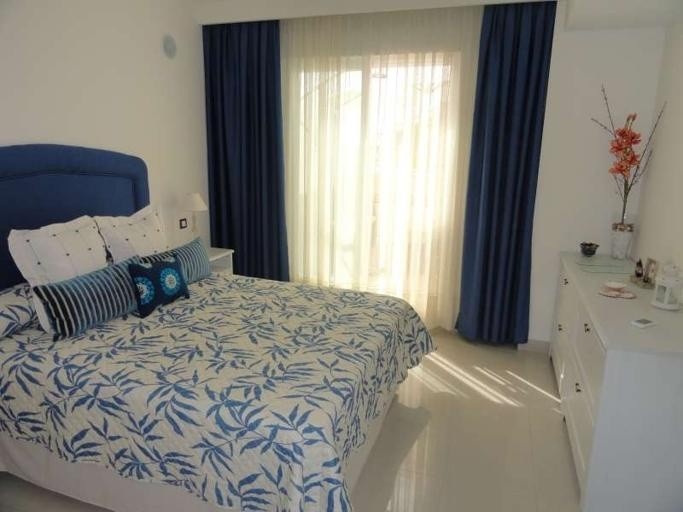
[548,252,683,512]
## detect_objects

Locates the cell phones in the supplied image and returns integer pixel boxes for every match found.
[632,317,656,329]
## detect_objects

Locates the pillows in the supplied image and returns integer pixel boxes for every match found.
[142,236,211,284]
[93,205,168,264]
[129,255,191,319]
[8,215,107,288]
[33,256,144,341]
[0,255,113,341]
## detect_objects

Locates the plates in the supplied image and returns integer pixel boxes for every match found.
[599,289,637,299]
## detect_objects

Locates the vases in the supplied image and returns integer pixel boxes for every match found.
[611,223,634,260]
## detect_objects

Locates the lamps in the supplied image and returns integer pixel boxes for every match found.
[177,193,208,231]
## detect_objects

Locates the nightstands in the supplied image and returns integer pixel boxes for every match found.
[210,247,235,275]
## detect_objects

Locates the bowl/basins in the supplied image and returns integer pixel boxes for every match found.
[603,282,627,290]
[580,242,601,256]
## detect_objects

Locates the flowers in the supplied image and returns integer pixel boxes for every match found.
[592,84,666,223]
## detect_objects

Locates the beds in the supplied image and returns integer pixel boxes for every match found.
[0,145,437,512]
